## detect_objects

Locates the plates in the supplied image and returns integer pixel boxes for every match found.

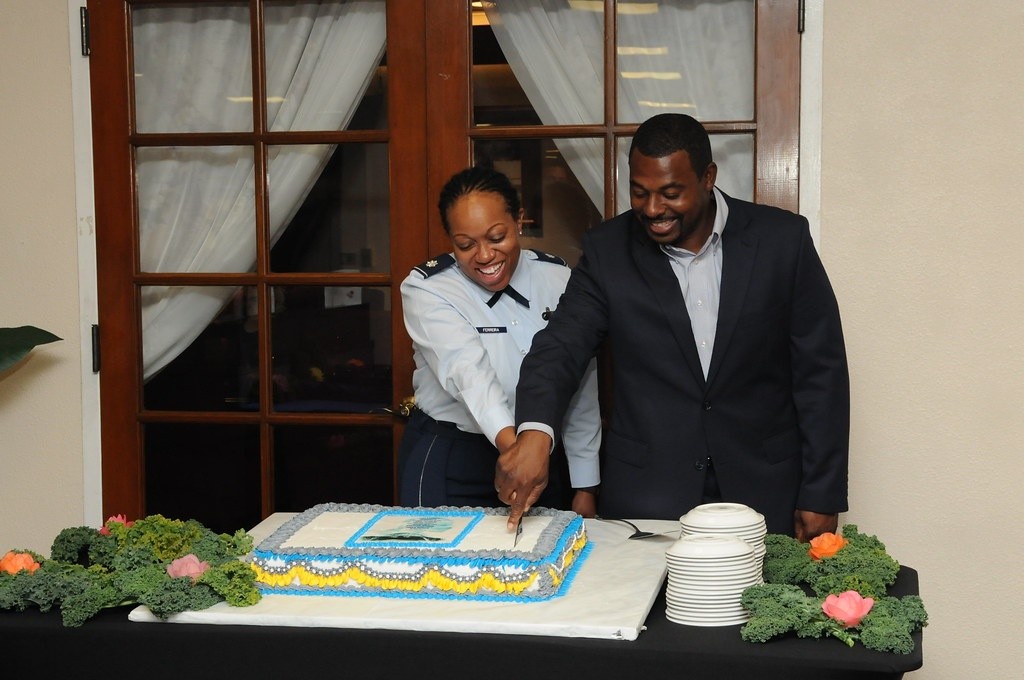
[665,502,768,627]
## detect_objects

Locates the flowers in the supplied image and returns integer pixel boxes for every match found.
[820,590,875,629]
[166,554,210,588]
[808,532,849,562]
[99,514,135,536]
[0,550,41,577]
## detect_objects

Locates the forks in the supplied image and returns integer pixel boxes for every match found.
[594,514,680,540]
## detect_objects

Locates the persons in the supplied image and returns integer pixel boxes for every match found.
[496,113,851,544]
[398,166,601,518]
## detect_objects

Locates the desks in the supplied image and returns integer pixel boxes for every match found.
[0,565,923,680]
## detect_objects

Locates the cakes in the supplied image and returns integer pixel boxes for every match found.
[244,503,591,601]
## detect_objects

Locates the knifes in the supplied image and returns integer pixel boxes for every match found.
[514,511,522,547]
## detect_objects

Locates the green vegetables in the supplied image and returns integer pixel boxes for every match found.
[0,512,263,627]
[740,524,928,654]
